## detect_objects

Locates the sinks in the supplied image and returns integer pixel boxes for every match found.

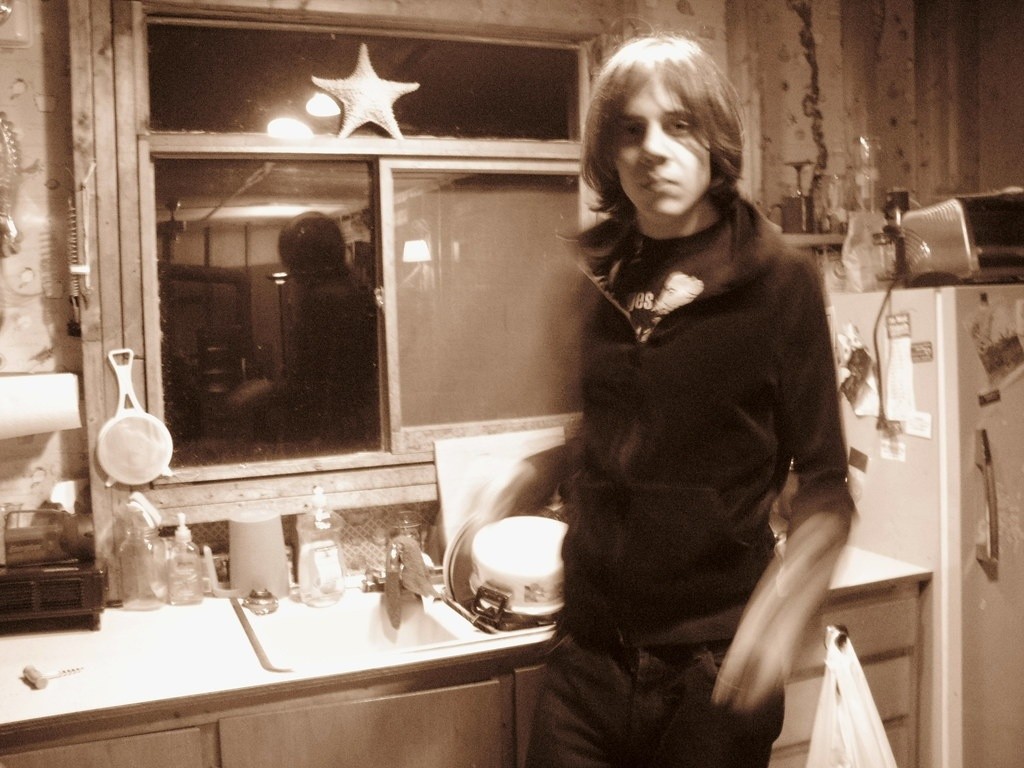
[228,591,461,674]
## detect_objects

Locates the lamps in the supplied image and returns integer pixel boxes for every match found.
[402,239,431,293]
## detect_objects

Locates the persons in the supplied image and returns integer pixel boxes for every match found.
[233,211,380,442]
[494,33,858,768]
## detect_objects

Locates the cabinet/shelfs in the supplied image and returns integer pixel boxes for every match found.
[0,665,545,768]
[767,585,920,768]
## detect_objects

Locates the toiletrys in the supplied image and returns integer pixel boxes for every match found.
[117,490,169,611]
[168,511,204,607]
[294,483,349,610]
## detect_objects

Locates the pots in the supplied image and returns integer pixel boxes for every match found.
[468,516,569,621]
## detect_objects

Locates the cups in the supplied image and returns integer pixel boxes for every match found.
[766,196,812,233]
[229,505,289,597]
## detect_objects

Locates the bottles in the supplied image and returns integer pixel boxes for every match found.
[295,486,345,607]
[118,528,167,611]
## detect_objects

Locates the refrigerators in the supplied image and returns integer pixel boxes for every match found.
[834,285,1024,768]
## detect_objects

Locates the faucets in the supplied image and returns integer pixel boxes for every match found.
[384,533,434,630]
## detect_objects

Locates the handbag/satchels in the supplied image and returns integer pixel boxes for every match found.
[804,628,898,768]
